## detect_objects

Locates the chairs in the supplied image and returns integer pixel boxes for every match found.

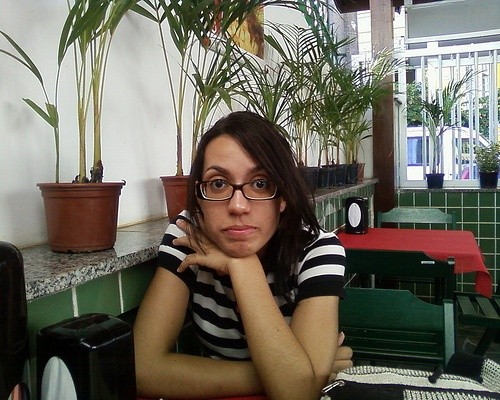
[377,206,455,293]
[325,283,456,375]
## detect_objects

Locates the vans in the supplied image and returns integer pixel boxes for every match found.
[407,126,497,180]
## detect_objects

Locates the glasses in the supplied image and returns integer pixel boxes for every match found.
[196,179,280,201]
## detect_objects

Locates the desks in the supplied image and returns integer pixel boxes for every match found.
[333,225,482,288]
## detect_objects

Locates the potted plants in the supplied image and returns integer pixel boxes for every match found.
[473,142,500,188]
[394,65,485,189]
[2,0,127,251]
[267,16,413,192]
[130,0,260,222]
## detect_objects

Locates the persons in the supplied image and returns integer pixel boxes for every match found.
[133,111,353,400]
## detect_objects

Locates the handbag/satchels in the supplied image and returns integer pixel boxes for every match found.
[320,352,499,400]
